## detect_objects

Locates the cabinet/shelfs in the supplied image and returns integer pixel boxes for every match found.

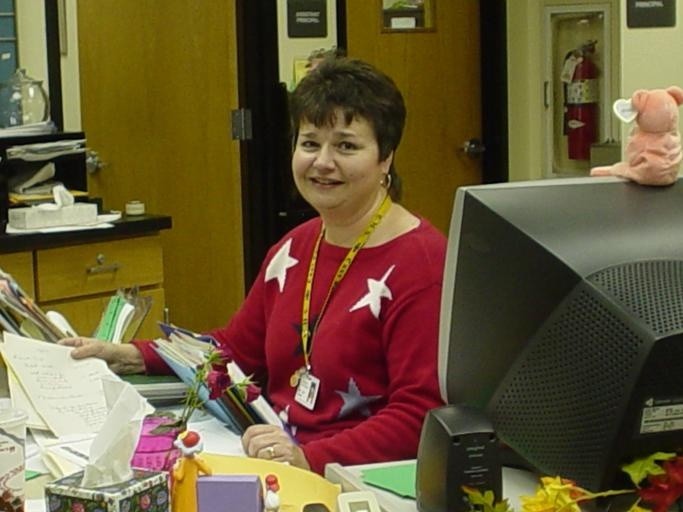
[1,231,166,344]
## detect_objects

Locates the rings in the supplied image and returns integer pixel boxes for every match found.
[267,446,276,457]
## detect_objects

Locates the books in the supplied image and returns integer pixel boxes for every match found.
[149,319,286,435]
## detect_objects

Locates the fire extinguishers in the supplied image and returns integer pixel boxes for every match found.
[561,38,600,161]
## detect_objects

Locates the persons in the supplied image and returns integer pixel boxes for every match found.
[306,385,314,403]
[55,54,447,477]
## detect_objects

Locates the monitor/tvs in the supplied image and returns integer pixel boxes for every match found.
[438,174,683,499]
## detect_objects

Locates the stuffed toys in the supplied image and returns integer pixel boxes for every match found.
[590,85,683,185]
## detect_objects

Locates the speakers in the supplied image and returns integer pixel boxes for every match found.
[415,406,502,512]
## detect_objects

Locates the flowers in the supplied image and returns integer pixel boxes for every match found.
[147,345,257,473]
